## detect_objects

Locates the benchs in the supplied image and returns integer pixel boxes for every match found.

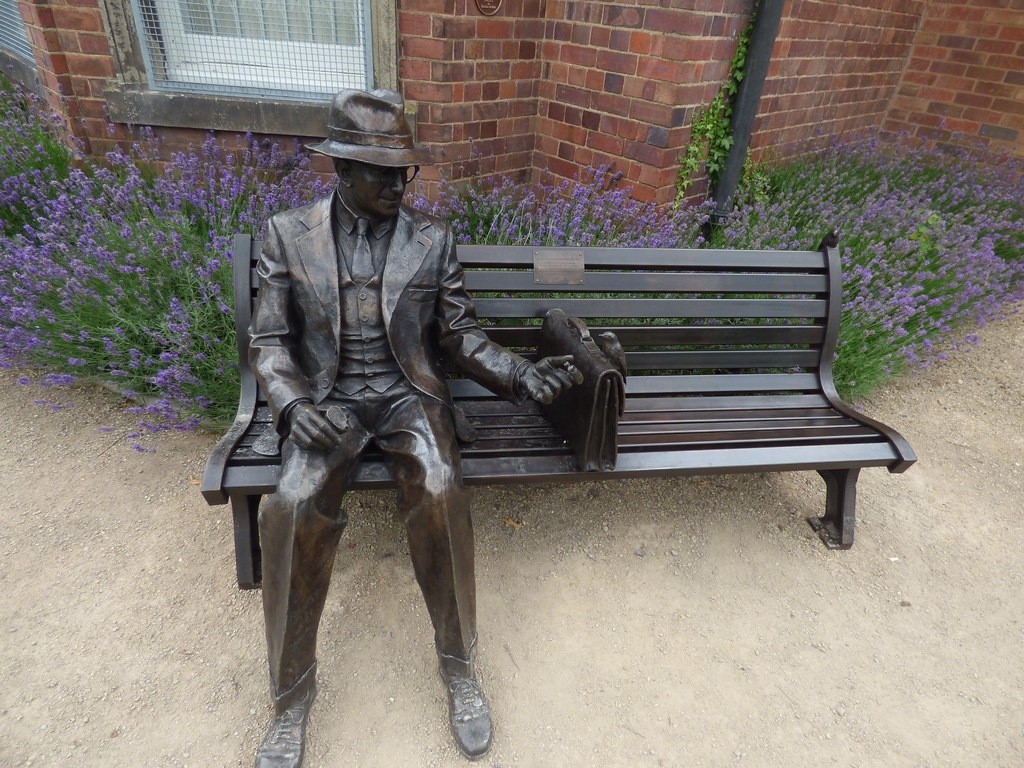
[199,228,917,592]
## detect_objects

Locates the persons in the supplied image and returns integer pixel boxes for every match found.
[249,90,585,768]
